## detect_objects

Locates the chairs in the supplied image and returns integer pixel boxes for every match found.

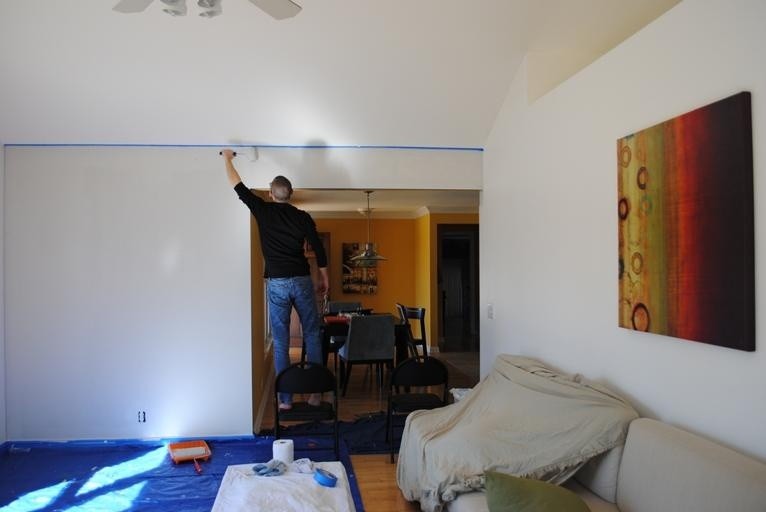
[272,302,449,464]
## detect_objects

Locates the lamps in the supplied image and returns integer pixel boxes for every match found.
[348,190,389,264]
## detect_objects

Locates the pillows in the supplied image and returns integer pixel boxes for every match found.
[482,468,590,512]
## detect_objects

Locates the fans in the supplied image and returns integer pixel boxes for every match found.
[113,1,304,22]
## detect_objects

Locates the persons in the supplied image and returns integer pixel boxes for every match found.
[222,148,332,410]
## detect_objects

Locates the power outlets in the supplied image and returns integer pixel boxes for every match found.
[136,410,147,424]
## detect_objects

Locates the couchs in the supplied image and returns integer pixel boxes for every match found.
[394,355,766,512]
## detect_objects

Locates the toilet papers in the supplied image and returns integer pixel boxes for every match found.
[272,440,294,467]
